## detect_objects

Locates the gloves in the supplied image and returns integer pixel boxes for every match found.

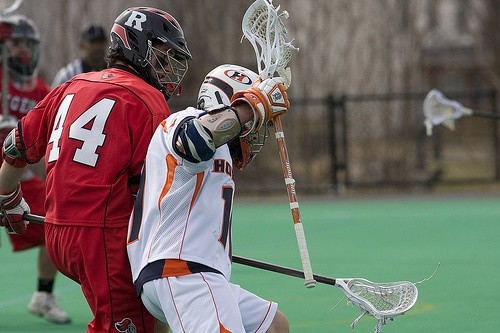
[0,182,32,237]
[231,75,291,134]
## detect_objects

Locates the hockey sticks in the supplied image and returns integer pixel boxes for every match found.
[0,0,24,122]
[422,87,500,126]
[20,212,420,324]
[240,0,318,289]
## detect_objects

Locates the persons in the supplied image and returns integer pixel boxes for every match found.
[127,64,290,333]
[0,7,192,333]
[0,15,70,322]
[51,25,108,89]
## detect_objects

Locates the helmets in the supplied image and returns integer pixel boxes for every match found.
[0,15,43,83]
[110,6,193,103]
[197,63,275,171]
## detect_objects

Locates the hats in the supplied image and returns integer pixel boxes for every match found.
[82,24,107,43]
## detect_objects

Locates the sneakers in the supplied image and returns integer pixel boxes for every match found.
[28,291,71,324]
